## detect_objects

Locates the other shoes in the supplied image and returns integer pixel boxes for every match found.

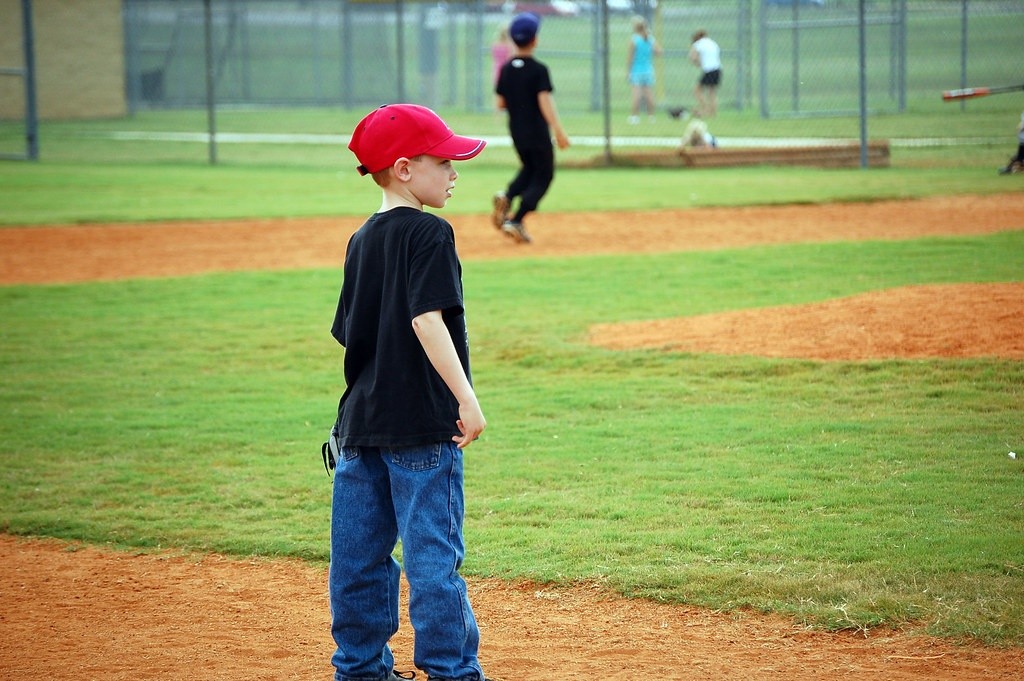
[501,222,533,243]
[384,669,416,681]
[492,192,511,229]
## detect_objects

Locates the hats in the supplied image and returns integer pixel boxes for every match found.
[511,10,541,45]
[348,104,486,176]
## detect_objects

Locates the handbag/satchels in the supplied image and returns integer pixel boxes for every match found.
[322,418,340,476]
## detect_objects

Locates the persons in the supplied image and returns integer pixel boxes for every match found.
[490,12,571,242]
[321,106,486,681]
[494,31,513,109]
[690,29,722,114]
[624,15,660,124]
